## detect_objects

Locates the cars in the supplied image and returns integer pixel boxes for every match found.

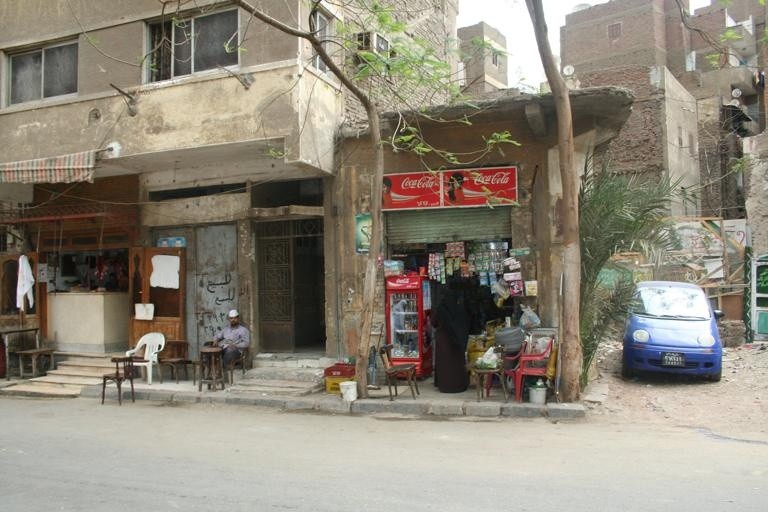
[622,280,726,383]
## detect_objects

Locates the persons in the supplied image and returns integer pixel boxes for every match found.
[432,292,470,393]
[206,309,250,380]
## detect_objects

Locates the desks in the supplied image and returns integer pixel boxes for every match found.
[1,328,41,381]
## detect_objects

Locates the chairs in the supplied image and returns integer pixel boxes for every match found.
[473,333,554,403]
[101,330,196,406]
[380,344,420,402]
[209,341,248,386]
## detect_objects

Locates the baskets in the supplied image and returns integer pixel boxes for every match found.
[324,364,357,394]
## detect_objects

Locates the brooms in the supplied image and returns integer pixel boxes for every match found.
[366,323,384,390]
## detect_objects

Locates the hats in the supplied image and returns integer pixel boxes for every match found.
[228,310,239,318]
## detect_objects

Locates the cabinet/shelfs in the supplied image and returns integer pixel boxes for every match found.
[129,246,188,359]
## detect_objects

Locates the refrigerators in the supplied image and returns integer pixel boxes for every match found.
[385,275,433,379]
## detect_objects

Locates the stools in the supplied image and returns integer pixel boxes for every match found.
[199,347,225,392]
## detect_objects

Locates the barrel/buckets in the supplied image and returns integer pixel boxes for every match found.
[528,386,548,405]
[338,380,357,402]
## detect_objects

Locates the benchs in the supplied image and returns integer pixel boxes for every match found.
[15,348,56,378]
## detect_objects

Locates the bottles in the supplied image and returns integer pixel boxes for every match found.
[393,293,417,312]
